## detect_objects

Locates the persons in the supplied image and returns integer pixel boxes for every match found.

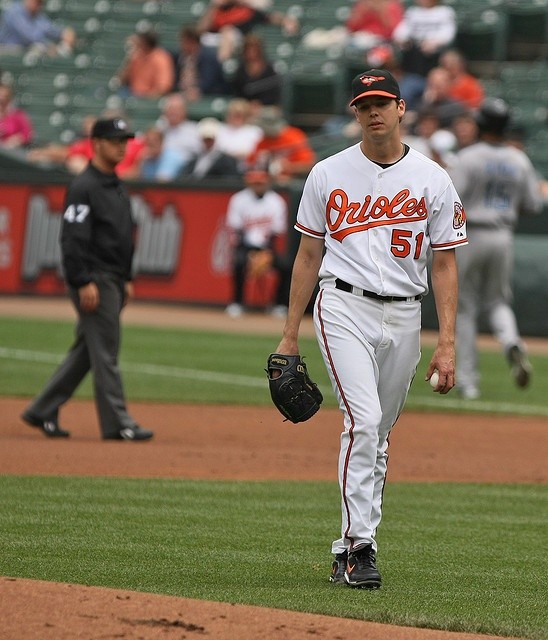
[393,1,458,74]
[110,33,174,100]
[244,34,281,106]
[216,99,264,169]
[196,0,299,61]
[28,116,162,179]
[1,2,75,57]
[177,116,238,183]
[178,30,216,94]
[22,119,154,439]
[226,170,291,318]
[332,0,403,67]
[440,99,547,397]
[142,94,194,181]
[408,110,456,169]
[0,86,30,146]
[247,111,316,175]
[426,50,479,109]
[272,68,467,586]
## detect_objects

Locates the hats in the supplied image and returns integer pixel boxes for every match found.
[91,117,134,139]
[348,69,400,108]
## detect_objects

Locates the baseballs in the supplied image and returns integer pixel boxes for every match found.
[429,373,439,388]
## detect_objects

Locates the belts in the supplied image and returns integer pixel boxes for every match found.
[335,278,420,301]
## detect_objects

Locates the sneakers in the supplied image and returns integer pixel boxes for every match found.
[329,548,346,583]
[507,344,529,387]
[343,542,380,587]
[106,424,152,440]
[19,410,69,437]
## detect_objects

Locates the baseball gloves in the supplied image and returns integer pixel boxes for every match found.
[264,353,323,424]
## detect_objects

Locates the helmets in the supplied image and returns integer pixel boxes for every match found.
[469,97,509,128]
